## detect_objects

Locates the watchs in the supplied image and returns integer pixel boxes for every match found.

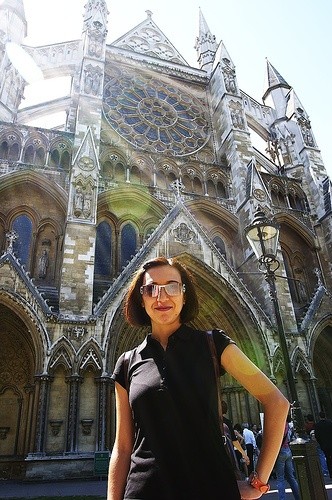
[248,471,270,495]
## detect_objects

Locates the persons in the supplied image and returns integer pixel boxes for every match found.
[38,250,49,281]
[276,416,300,500]
[305,411,332,479]
[220,400,250,481]
[234,422,263,483]
[107,256,291,500]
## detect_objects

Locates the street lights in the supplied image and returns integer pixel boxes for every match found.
[243,204,329,500]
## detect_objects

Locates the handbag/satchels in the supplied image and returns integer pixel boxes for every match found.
[222,435,248,480]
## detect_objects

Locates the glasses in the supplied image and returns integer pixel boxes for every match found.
[139,284,186,297]
